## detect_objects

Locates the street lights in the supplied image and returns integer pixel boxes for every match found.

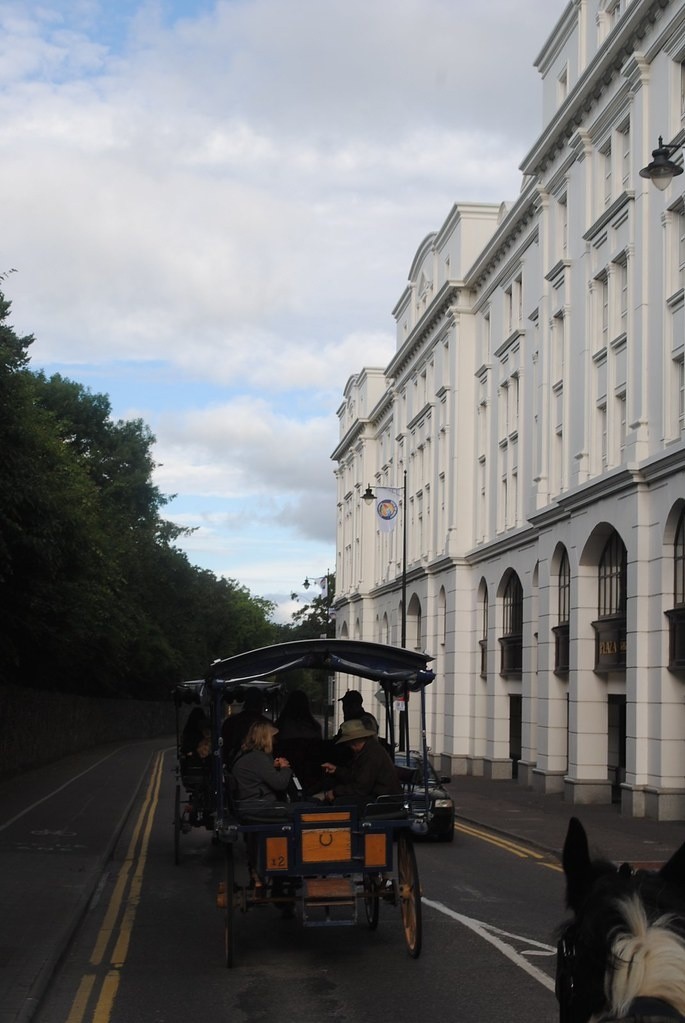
[359,470,406,752]
[302,569,330,740]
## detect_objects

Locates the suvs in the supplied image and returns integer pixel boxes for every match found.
[395,750,455,844]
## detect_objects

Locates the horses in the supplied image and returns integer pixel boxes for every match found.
[556,815,685,1023]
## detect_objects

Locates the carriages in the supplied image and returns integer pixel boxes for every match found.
[166,638,437,970]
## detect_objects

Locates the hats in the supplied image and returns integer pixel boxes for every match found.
[336,719,376,743]
[338,690,363,701]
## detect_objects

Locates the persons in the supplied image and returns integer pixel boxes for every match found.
[179,686,404,805]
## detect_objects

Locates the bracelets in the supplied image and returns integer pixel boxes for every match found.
[324,791,330,801]
